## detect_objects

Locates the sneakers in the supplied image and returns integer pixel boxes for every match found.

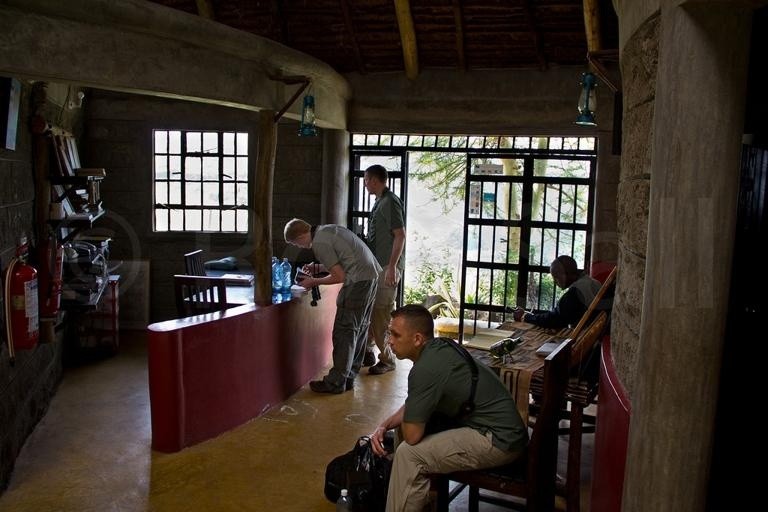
[361,352,376,367]
[368,359,397,375]
[309,379,354,394]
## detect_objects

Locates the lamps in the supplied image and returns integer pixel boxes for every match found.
[577,50,620,126]
[68,92,84,109]
[270,75,317,136]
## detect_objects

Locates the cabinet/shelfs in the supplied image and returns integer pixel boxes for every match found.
[32,120,109,311]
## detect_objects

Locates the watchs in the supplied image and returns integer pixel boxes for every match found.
[520,312,527,322]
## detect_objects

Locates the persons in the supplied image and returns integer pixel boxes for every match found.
[284,165,406,394]
[369,304,530,512]
[513,255,613,341]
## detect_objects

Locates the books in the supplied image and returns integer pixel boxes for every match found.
[45,134,82,178]
[464,328,515,351]
[536,343,562,356]
[222,274,255,286]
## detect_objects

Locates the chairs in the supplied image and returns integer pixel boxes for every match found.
[172,274,226,318]
[437,339,572,512]
[184,249,207,276]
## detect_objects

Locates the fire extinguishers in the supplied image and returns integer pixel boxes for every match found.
[5,231,40,368]
[33,222,65,319]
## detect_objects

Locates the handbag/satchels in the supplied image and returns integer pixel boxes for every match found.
[324,436,392,512]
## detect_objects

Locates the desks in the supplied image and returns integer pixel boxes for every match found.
[460,322,598,512]
[183,265,254,313]
[81,275,120,354]
[148,281,347,454]
[185,284,254,314]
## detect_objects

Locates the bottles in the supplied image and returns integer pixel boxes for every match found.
[335,486,351,510]
[489,336,524,359]
[272,257,292,291]
[273,293,292,302]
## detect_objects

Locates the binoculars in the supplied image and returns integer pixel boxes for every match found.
[311,286,321,301]
